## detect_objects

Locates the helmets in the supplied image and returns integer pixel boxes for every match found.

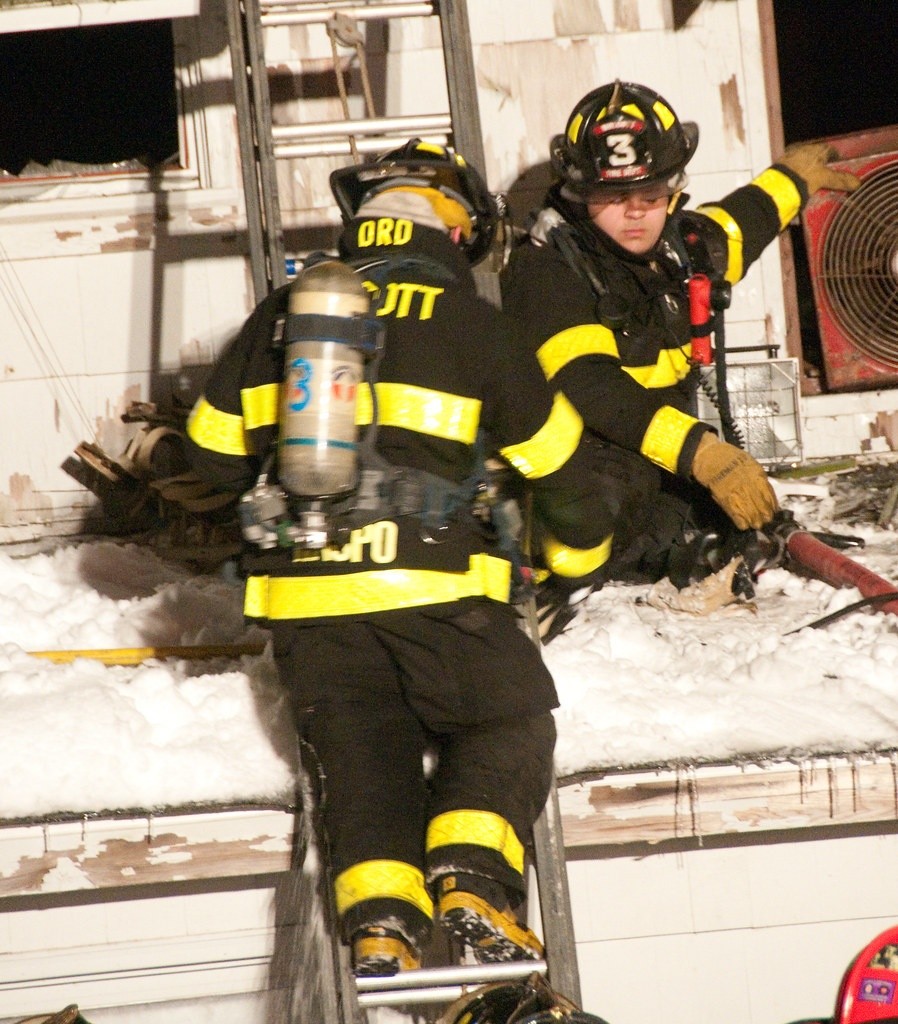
[330,141,499,270]
[550,80,700,194]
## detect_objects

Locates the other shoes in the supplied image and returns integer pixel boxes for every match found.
[350,925,424,979]
[437,873,547,981]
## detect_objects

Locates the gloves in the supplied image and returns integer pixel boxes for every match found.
[775,139,860,199]
[690,431,779,530]
[529,567,606,645]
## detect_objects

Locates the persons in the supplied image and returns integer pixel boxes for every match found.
[490,80,861,605]
[137,139,613,977]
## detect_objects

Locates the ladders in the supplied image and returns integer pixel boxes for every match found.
[220,0,584,1024]
